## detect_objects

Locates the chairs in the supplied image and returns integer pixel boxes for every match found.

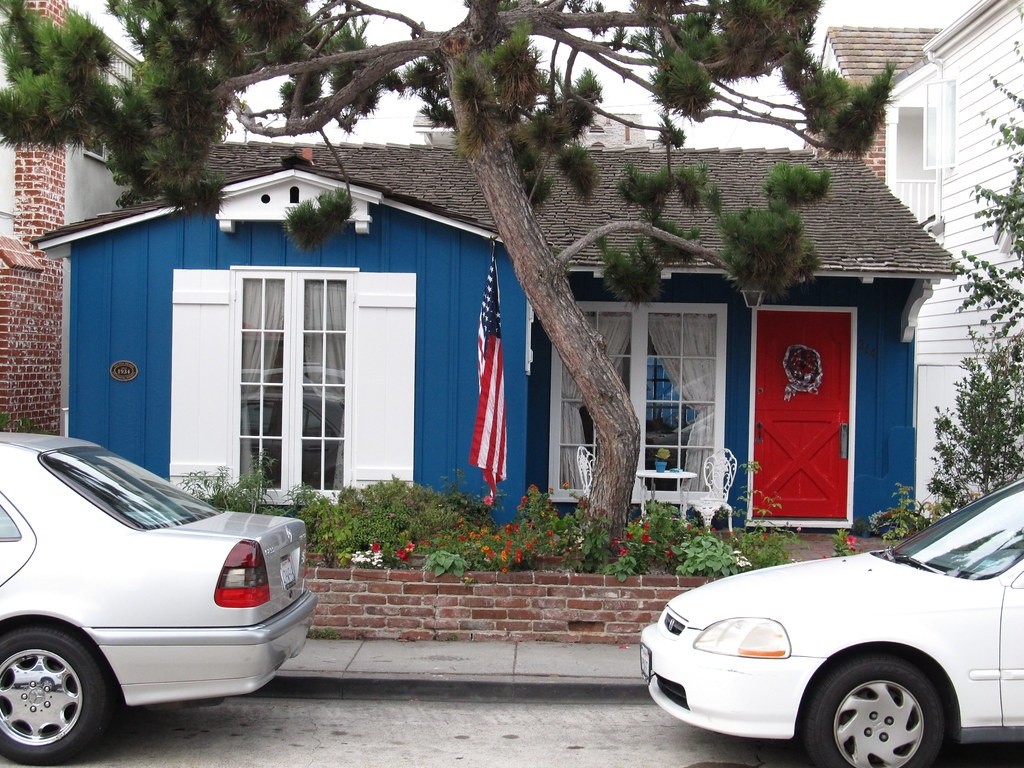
[686,449,738,535]
[576,446,596,502]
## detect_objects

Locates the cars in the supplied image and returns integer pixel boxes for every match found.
[0,431,318,766]
[639,475,1024,767]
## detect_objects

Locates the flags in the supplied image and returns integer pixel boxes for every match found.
[468,252,509,501]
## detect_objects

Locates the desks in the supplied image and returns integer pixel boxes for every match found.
[635,469,697,525]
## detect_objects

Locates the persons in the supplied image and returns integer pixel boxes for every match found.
[672,410,700,436]
[580,404,658,457]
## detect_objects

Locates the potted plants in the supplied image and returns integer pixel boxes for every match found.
[654,448,670,472]
